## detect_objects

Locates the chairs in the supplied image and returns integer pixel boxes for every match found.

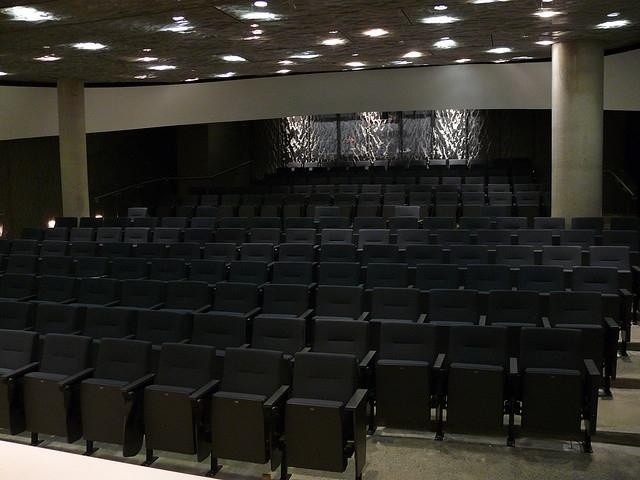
[1,161,639,479]
